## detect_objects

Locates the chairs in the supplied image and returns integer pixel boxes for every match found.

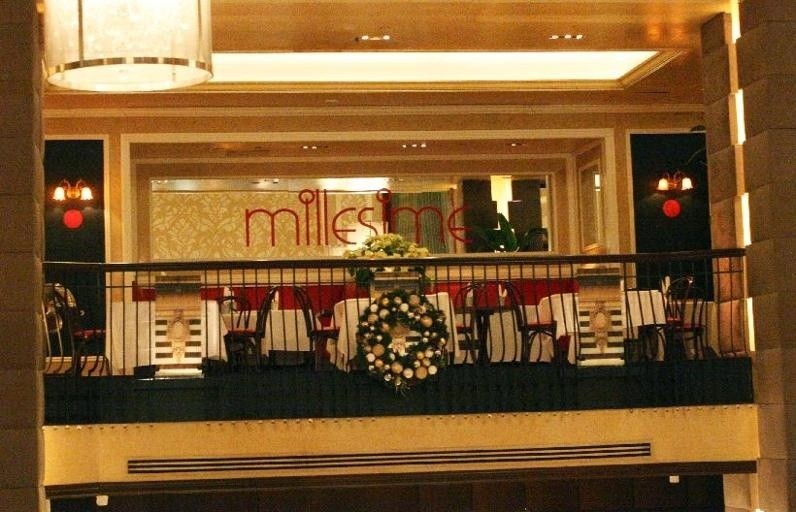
[42,283,105,377]
[216,276,708,370]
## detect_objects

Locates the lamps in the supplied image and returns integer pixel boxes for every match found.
[45,0,214,94]
[52,179,94,203]
[656,169,694,198]
